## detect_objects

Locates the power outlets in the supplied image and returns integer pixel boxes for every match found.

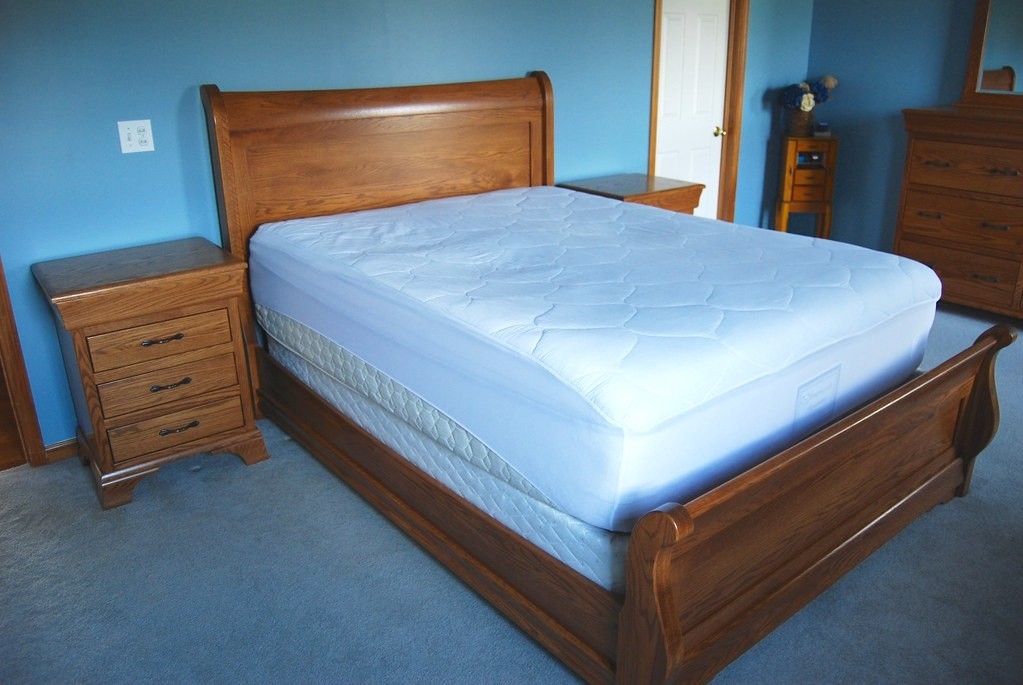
[117,119,156,154]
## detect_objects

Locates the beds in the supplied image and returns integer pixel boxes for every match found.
[200,72,1018,685]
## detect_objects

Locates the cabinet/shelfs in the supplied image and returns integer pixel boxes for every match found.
[777,135,838,203]
[891,104,1023,319]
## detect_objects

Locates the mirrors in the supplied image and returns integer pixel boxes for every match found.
[960,0,1023,108]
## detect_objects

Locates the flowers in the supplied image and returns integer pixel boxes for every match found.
[777,75,839,112]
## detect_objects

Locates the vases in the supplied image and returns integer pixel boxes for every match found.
[788,109,815,137]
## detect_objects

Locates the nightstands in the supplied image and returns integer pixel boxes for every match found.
[30,234,270,510]
[557,173,707,214]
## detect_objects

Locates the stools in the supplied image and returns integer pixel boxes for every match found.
[775,196,833,240]
